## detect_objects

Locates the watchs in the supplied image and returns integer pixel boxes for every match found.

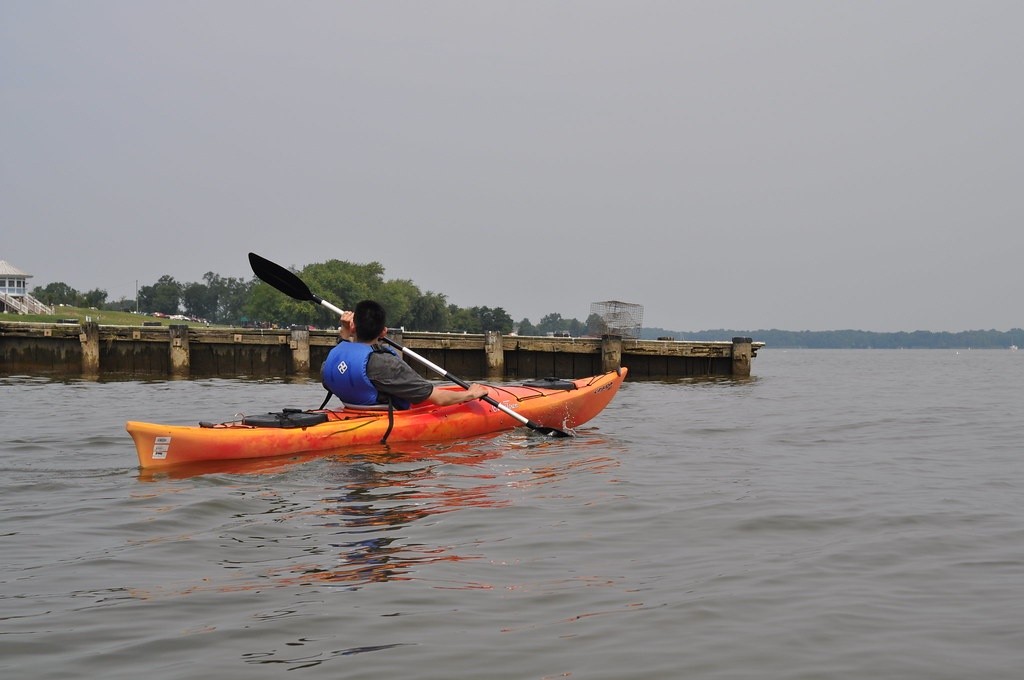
[336,336,351,344]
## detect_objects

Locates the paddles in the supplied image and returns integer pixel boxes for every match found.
[248,250,574,439]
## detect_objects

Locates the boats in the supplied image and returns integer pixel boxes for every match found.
[126,366,627,477]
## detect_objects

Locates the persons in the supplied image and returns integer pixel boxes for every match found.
[320,299,488,411]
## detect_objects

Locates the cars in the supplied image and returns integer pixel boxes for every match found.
[154,312,201,323]
